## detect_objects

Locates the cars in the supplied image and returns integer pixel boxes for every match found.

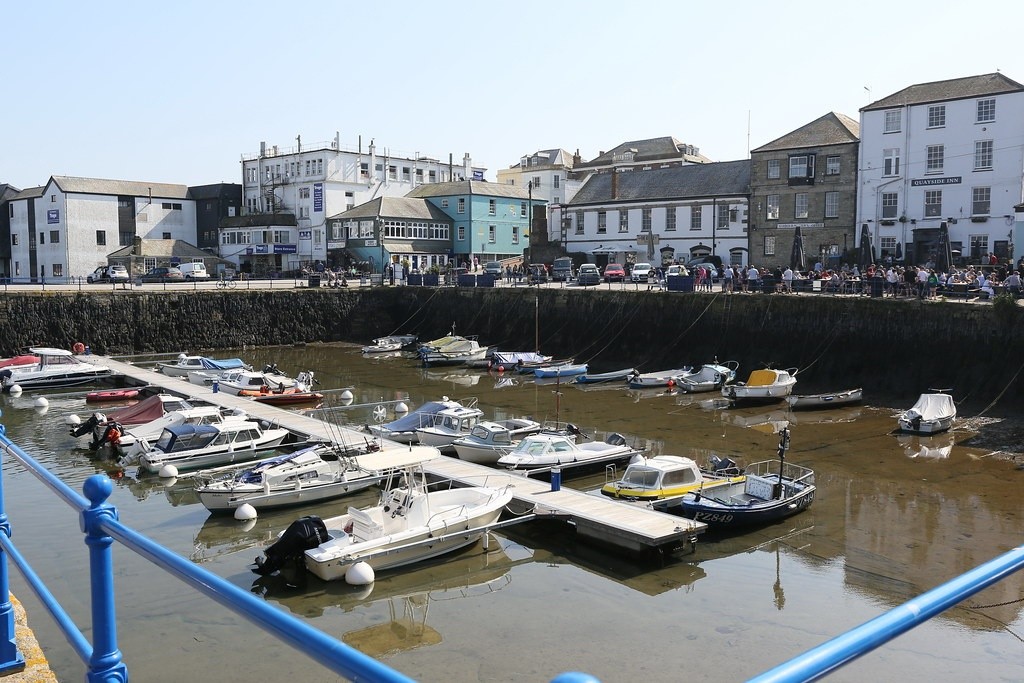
[483,261,503,279]
[695,263,718,282]
[629,262,654,283]
[138,267,183,281]
[527,263,548,281]
[665,265,690,284]
[444,268,469,284]
[604,264,626,281]
[87,264,129,284]
[578,264,600,285]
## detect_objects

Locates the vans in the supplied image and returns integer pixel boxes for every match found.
[176,262,207,280]
[552,257,577,280]
[686,256,723,274]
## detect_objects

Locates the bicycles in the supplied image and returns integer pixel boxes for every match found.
[216,277,237,289]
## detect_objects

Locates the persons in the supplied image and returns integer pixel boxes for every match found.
[678,252,1024,300]
[473,256,478,272]
[506,263,512,283]
[647,267,665,291]
[667,379,674,391]
[513,264,517,283]
[517,265,524,282]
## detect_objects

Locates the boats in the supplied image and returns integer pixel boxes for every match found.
[69,393,516,584]
[156,353,324,402]
[897,389,956,432]
[0,346,112,391]
[574,356,864,407]
[365,388,650,477]
[362,333,419,354]
[88,389,139,399]
[588,425,817,534]
[411,332,589,376]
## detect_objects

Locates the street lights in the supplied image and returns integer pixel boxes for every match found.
[379,229,384,285]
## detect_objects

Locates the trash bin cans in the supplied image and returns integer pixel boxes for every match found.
[423,274,438,286]
[458,275,475,287]
[408,274,422,285]
[309,276,320,287]
[477,275,494,287]
[668,276,694,291]
